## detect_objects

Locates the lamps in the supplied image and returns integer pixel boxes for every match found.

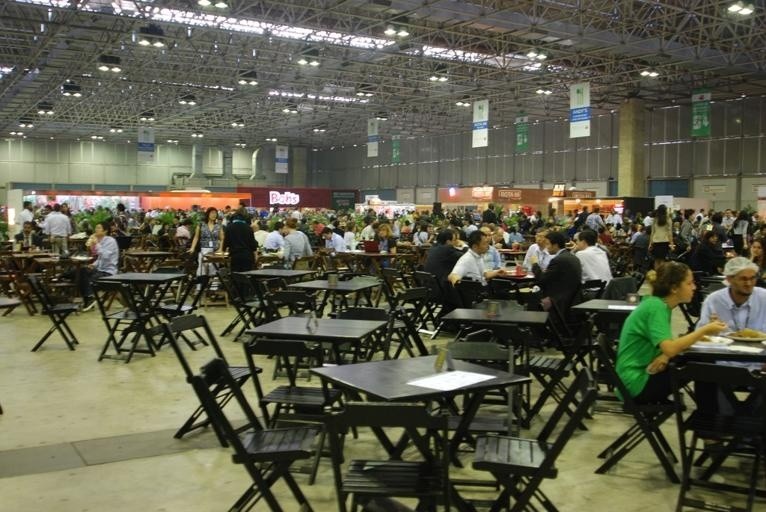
[313,122,328,133]
[382,13,410,38]
[97,54,123,73]
[534,76,553,96]
[638,60,661,78]
[296,45,322,67]
[375,111,389,121]
[191,127,204,138]
[235,138,246,146]
[62,79,82,97]
[282,99,298,114]
[139,109,156,122]
[727,1,756,17]
[37,101,54,116]
[428,62,448,82]
[238,68,258,86]
[265,134,278,142]
[456,94,472,108]
[356,82,374,97]
[137,22,165,48]
[178,91,197,105]
[109,119,124,133]
[17,113,36,127]
[526,39,549,60]
[231,116,246,128]
[195,0,232,11]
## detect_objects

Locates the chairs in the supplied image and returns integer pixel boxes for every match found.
[471,366,599,511]
[1,256,39,317]
[676,360,766,512]
[187,357,321,511]
[527,319,595,431]
[433,340,522,493]
[591,333,684,485]
[159,314,263,449]
[678,270,706,332]
[596,277,639,329]
[90,280,156,363]
[215,271,285,341]
[486,280,521,301]
[365,287,433,359]
[333,305,389,405]
[155,274,211,351]
[453,277,486,339]
[275,256,324,322]
[399,270,444,331]
[545,279,608,349]
[26,275,82,351]
[321,401,453,512]
[245,338,345,485]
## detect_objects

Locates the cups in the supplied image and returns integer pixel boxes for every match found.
[516,265,522,275]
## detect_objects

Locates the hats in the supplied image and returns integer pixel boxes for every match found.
[723,257,759,280]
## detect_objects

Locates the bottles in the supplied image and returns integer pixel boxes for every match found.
[85,232,97,248]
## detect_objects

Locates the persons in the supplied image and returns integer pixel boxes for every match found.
[44,203,73,260]
[344,222,360,249]
[696,216,702,223]
[677,208,700,260]
[706,208,739,231]
[220,205,231,219]
[326,205,349,235]
[531,232,584,328]
[14,221,43,249]
[298,218,310,234]
[262,222,284,255]
[129,203,199,249]
[691,230,727,280]
[223,206,259,302]
[186,207,224,311]
[648,204,676,270]
[673,209,684,225]
[284,216,314,281]
[696,209,705,220]
[312,221,325,236]
[731,210,750,256]
[693,255,765,450]
[320,227,347,262]
[613,261,731,457]
[478,226,503,271]
[423,229,465,333]
[36,203,52,228]
[574,228,613,302]
[15,201,34,230]
[109,202,132,249]
[522,227,557,273]
[79,221,120,312]
[673,222,683,246]
[447,230,510,303]
[258,203,302,223]
[372,224,398,302]
[69,205,111,230]
[749,238,766,287]
[751,211,765,239]
[691,222,699,237]
[477,203,654,253]
[253,224,269,246]
[61,203,78,235]
[360,208,476,248]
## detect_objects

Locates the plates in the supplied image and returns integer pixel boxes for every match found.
[694,337,733,346]
[725,332,766,341]
[507,274,527,277]
